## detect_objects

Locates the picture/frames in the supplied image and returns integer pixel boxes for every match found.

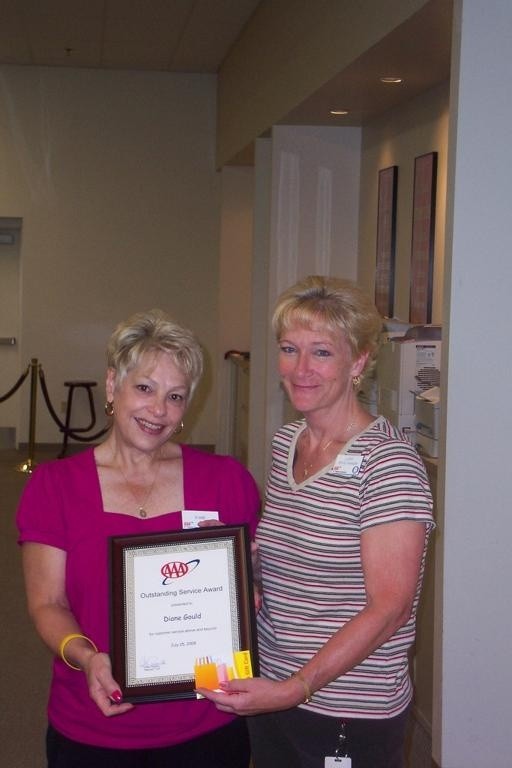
[108,523,260,705]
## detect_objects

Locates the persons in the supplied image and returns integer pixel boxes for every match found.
[191,274,441,767]
[9,308,264,768]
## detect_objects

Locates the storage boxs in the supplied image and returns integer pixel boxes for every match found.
[358,318,442,458]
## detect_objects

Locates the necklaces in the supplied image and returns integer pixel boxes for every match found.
[300,407,363,479]
[106,438,166,521]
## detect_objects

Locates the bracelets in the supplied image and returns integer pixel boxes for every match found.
[290,670,312,704]
[57,633,100,671]
